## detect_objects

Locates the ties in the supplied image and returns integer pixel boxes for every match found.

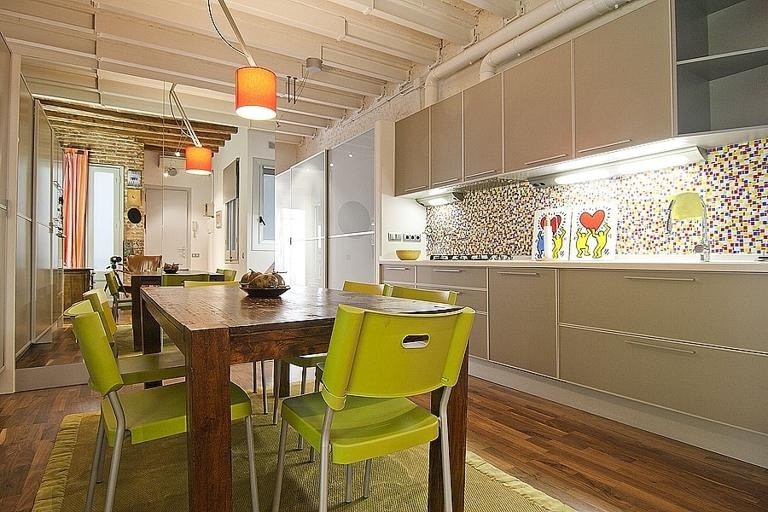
[222,157,239,204]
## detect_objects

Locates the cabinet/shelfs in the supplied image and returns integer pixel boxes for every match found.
[416,265,486,289]
[673,0,766,63]
[429,90,463,196]
[15,73,65,365]
[274,128,375,291]
[379,264,415,288]
[676,48,768,148]
[487,266,556,379]
[468,313,488,360]
[503,38,577,182]
[558,325,766,435]
[462,73,503,183]
[395,105,430,199]
[573,2,709,166]
[558,267,766,354]
[416,286,487,313]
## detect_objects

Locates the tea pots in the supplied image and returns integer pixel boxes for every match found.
[9,73,276,391]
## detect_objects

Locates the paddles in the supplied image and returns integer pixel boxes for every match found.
[33,380,579,512]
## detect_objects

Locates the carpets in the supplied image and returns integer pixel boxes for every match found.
[33,380,579,512]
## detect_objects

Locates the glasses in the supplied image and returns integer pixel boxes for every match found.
[164,79,212,177]
[204,0,278,123]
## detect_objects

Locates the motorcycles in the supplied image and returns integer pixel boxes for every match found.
[104,269,128,298]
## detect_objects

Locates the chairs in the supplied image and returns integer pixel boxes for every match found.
[63,300,259,512]
[126,254,163,273]
[253,279,459,503]
[105,270,132,323]
[272,304,475,511]
[161,269,239,288]
[82,287,186,484]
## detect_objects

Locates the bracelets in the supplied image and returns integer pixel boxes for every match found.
[239,286,290,297]
[164,269,178,273]
[396,250,421,260]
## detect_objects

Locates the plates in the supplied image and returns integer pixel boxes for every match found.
[15,73,65,365]
[274,128,375,291]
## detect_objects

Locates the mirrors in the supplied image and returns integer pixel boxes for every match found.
[9,73,276,391]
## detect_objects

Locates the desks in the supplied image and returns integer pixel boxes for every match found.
[131,271,225,352]
[140,286,469,512]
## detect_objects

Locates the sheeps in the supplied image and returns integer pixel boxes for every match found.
[665,193,710,262]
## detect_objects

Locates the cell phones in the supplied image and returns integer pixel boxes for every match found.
[110,256,122,263]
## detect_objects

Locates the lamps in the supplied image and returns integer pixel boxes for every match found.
[204,0,278,123]
[126,254,163,273]
[164,79,212,177]
